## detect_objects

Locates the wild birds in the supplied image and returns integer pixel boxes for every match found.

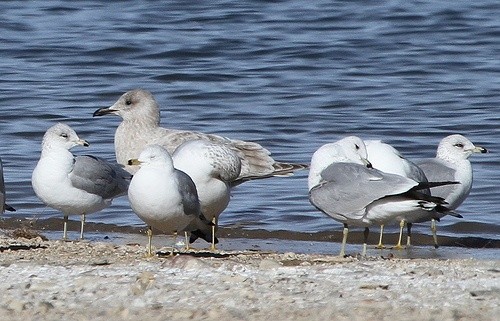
[31,89,488,262]
